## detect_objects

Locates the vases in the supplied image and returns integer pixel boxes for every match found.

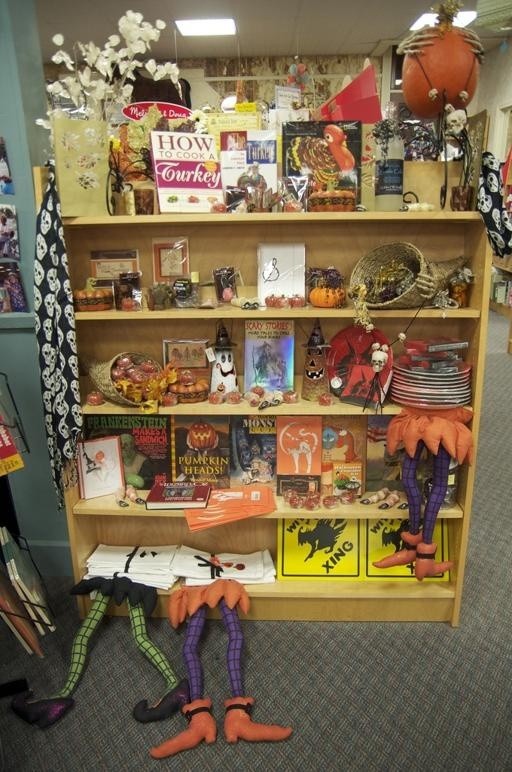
[53,118,109,218]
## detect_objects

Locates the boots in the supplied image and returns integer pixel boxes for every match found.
[224,697,294,744]
[415,541,454,581]
[371,533,423,568]
[149,698,218,759]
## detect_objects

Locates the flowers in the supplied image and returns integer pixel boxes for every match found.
[47,9,172,118]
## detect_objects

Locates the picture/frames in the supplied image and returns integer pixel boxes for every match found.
[91,257,139,279]
[153,242,189,282]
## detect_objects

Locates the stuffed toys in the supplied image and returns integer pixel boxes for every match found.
[150,576,295,760]
[12,577,193,729]
[387,405,475,580]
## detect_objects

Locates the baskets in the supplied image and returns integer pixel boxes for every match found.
[80,350,164,407]
[349,243,474,311]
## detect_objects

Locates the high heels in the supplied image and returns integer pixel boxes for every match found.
[133,679,192,724]
[11,696,76,730]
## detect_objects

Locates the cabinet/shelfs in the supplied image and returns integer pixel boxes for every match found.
[31,161,498,629]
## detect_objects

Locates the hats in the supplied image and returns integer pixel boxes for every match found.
[209,319,238,349]
[301,318,332,349]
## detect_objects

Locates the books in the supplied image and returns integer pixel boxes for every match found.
[145,481,213,510]
[148,131,227,213]
[84,416,170,489]
[0,403,25,480]
[235,416,276,487]
[339,364,394,410]
[173,416,233,490]
[324,416,366,495]
[368,417,449,493]
[279,121,362,213]
[1,525,57,635]
[243,318,296,394]
[0,590,44,660]
[278,416,321,476]
[220,128,282,212]
[333,461,364,496]
